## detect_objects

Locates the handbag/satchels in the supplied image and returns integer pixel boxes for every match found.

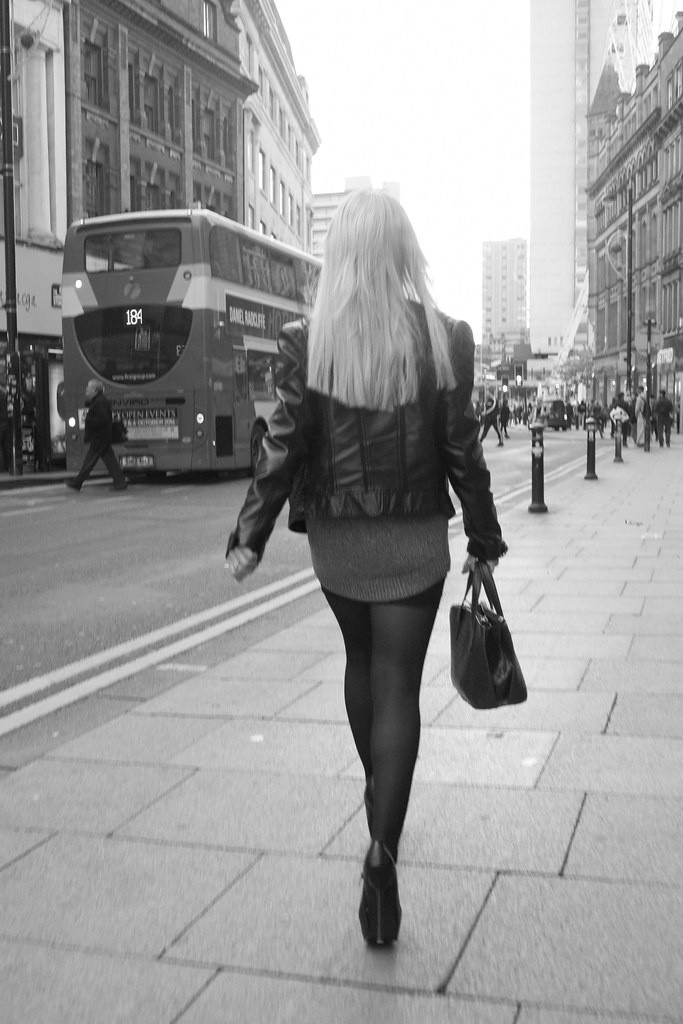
[449,558,528,710]
[111,421,127,443]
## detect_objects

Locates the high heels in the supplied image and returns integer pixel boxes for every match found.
[359,842,402,947]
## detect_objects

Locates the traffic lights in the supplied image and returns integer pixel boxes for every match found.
[500,378,509,394]
[513,364,524,389]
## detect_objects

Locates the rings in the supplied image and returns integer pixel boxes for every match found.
[222,562,230,569]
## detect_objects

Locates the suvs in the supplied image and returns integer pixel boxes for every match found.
[534,399,568,432]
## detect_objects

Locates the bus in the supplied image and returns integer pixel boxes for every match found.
[59,210,327,482]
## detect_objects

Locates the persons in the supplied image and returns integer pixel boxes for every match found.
[63,379,129,492]
[499,399,510,439]
[512,387,674,449]
[479,388,505,447]
[221,190,509,950]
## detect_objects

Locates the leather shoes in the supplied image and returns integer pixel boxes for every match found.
[109,479,127,491]
[64,478,82,493]
